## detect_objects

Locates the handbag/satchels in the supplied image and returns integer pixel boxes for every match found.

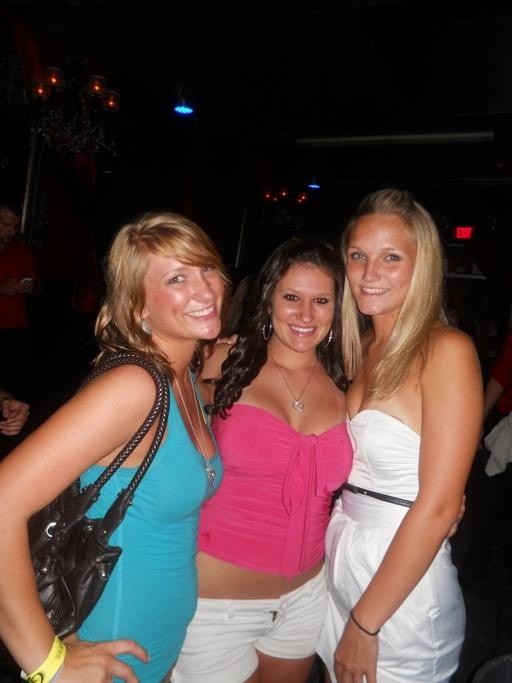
[27,351,170,642]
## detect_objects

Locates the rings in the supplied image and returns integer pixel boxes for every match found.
[20,409,31,416]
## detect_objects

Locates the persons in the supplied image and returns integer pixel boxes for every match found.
[313,186,486,683]
[0,195,39,401]
[0,387,31,439]
[168,237,468,683]
[0,214,242,683]
[469,323,511,584]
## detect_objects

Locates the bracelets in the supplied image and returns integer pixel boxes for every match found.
[347,610,380,635]
[18,635,67,682]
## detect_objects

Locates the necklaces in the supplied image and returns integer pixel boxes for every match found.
[267,350,320,411]
[166,363,216,486]
